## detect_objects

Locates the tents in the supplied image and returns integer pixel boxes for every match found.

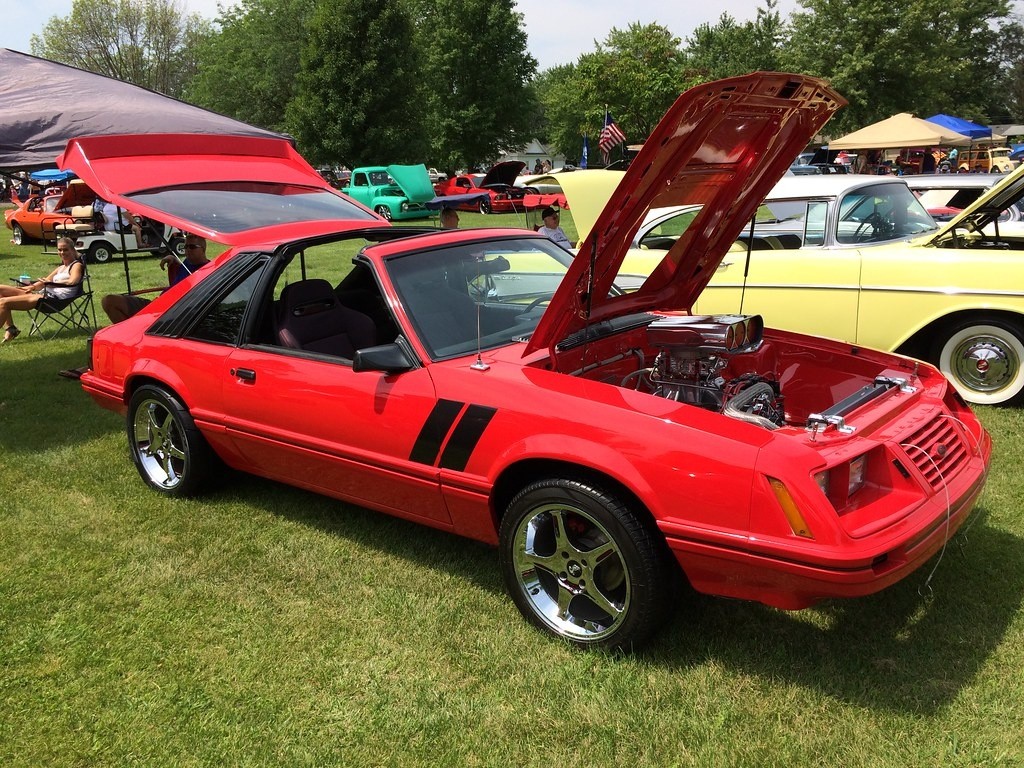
[824,113,973,174]
[972,132,1008,145]
[0,48,296,292]
[923,114,995,173]
[29,169,79,198]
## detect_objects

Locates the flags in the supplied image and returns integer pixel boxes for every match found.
[599,108,627,167]
[581,132,588,169]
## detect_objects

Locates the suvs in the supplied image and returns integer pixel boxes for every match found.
[958,147,1024,174]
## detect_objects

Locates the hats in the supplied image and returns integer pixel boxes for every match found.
[542,207,560,220]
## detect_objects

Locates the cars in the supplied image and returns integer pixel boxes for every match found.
[4,180,96,246]
[433,160,535,216]
[789,145,846,176]
[479,163,1024,408]
[336,165,441,222]
[834,154,859,174]
[315,169,352,189]
[57,73,992,656]
[740,165,1024,237]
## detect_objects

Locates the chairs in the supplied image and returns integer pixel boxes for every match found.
[727,236,773,251]
[762,234,808,250]
[375,269,459,342]
[259,279,374,356]
[940,165,951,175]
[8,253,97,341]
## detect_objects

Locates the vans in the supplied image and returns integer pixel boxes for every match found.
[427,167,447,183]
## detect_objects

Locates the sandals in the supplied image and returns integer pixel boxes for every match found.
[0,325,21,343]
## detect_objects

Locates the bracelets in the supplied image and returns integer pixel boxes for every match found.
[32,284,36,291]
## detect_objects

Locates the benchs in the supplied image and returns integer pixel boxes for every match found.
[55,205,94,230]
[95,211,133,235]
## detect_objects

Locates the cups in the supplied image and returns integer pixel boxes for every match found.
[19,276,31,286]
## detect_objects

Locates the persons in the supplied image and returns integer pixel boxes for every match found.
[834,146,984,175]
[538,207,573,250]
[524,167,531,175]
[0,172,57,204]
[440,208,460,228]
[104,201,150,248]
[0,237,85,344]
[57,235,212,379]
[534,159,551,176]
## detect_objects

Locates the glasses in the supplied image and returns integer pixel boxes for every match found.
[184,244,205,250]
